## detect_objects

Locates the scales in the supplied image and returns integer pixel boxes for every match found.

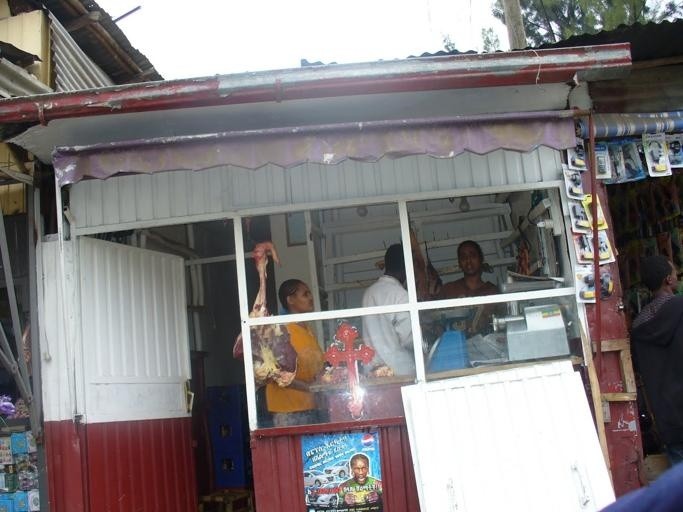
[425,308,470,374]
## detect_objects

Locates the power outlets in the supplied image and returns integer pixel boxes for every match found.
[544,218,554,229]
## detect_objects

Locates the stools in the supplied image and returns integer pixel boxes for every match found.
[198,488,255,512]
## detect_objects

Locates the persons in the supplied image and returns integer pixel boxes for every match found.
[263,278,326,428]
[359,242,436,385]
[336,452,383,509]
[629,253,683,468]
[419,239,510,341]
[305,486,320,504]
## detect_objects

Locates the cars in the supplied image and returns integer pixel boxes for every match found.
[304,460,351,510]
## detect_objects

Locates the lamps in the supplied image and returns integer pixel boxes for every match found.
[459,196,469,212]
[357,206,368,217]
